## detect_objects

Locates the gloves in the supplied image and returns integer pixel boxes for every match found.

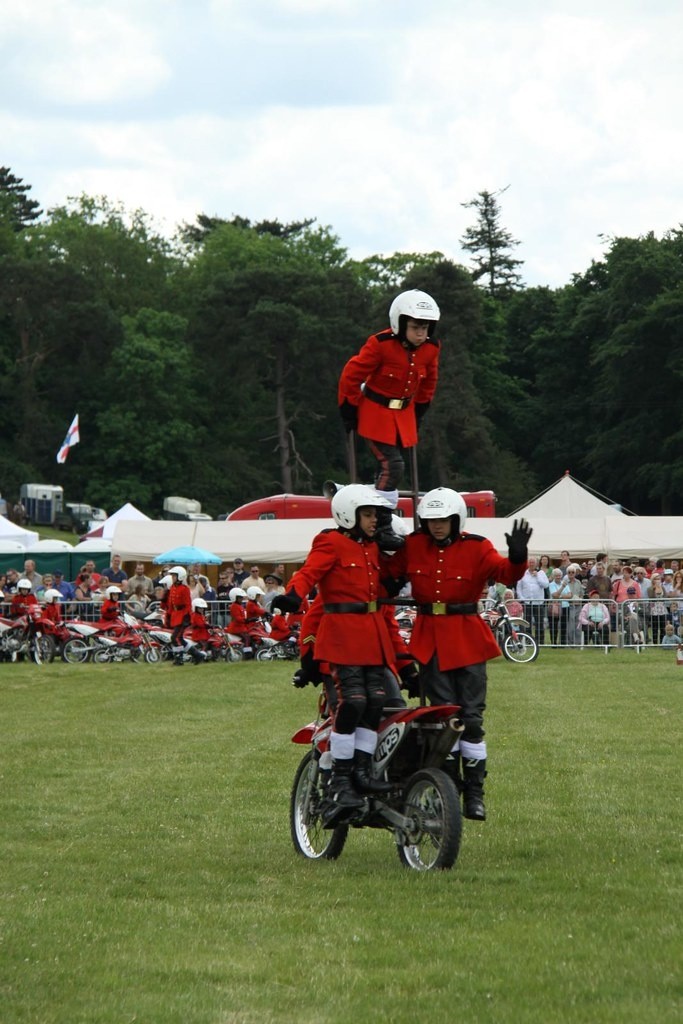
[291,655,313,688]
[269,586,303,616]
[340,396,358,434]
[398,662,422,700]
[504,517,533,565]
[415,401,431,432]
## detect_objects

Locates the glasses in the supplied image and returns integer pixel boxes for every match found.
[219,578,226,580]
[568,572,574,574]
[251,570,258,573]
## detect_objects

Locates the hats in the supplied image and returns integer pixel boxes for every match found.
[663,569,673,574]
[630,557,639,563]
[588,590,598,597]
[53,568,63,577]
[234,558,242,563]
[627,586,636,594]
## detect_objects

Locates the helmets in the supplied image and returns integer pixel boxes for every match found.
[106,585,122,600]
[247,586,265,600]
[389,289,440,335]
[331,483,467,535]
[16,578,63,603]
[159,566,208,613]
[229,587,247,603]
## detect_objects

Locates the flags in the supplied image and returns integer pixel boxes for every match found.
[57,414,81,464]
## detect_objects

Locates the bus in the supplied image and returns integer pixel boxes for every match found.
[227,489,497,520]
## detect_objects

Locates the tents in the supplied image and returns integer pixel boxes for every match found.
[0,472,683,563]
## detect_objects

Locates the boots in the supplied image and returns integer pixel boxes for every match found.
[376,488,410,556]
[172,643,205,666]
[444,738,489,820]
[328,729,392,807]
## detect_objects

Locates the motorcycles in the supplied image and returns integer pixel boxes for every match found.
[478,596,540,664]
[0,603,417,664]
[290,674,464,873]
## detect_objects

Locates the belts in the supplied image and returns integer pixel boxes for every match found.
[363,384,413,410]
[323,597,478,614]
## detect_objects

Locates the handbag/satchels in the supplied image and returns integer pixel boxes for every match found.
[547,597,561,617]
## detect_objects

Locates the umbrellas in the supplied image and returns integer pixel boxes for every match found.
[153,545,222,580]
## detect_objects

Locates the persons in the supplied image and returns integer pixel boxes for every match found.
[339,288,441,546]
[0,485,683,821]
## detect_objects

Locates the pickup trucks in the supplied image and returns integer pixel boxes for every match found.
[55,504,96,535]
